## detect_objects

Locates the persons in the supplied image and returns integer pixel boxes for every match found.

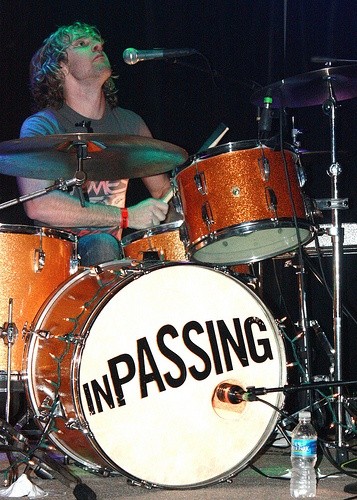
[14,24,188,266]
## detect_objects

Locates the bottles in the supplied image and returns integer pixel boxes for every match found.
[290,411,318,498]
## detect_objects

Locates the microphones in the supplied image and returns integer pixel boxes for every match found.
[216,383,259,404]
[258,85,276,133]
[123,48,196,64]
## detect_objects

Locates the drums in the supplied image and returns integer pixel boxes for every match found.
[118,219,259,292]
[269,149,357,261]
[171,136,321,267]
[22,256,290,492]
[0,222,80,395]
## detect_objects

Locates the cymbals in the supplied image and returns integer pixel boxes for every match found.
[247,63,357,110]
[0,131,189,182]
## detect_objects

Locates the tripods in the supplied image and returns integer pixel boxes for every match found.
[277,71,357,467]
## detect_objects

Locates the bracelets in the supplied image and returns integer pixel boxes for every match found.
[120,207,128,229]
